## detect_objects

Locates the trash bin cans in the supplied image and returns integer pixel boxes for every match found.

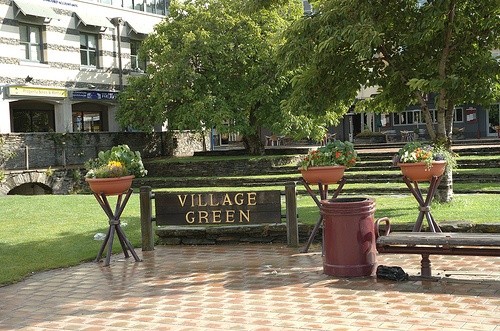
[321,197,390,278]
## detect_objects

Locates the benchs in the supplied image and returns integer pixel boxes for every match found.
[374,216,500,279]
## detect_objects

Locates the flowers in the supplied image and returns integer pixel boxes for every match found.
[297,140,360,168]
[84,145,148,178]
[398,141,453,166]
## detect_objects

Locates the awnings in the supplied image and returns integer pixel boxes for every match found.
[14,0,60,23]
[74,11,115,32]
[125,17,156,34]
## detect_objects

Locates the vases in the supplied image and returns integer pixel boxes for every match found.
[399,162,448,183]
[299,165,344,184]
[85,176,135,194]
